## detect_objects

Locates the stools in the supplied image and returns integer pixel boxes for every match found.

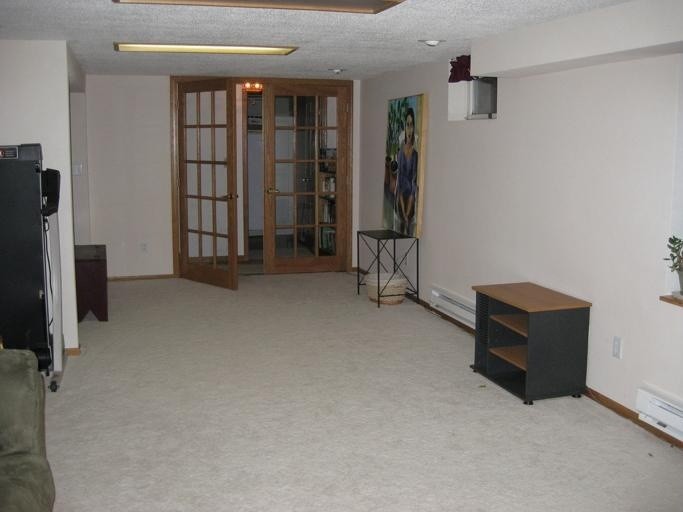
[74,244,108,322]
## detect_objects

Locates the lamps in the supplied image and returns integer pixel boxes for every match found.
[245,82,263,92]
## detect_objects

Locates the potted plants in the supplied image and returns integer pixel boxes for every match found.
[662,235,683,295]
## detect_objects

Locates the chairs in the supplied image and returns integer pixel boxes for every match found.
[0,349,55,512]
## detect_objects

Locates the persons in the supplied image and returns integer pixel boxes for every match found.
[392,107,418,236]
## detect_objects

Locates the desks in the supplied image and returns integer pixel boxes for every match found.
[357,230,419,308]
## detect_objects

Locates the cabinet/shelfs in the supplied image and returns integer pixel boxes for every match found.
[469,281,592,405]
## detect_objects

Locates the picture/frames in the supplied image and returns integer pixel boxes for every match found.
[383,93,430,239]
